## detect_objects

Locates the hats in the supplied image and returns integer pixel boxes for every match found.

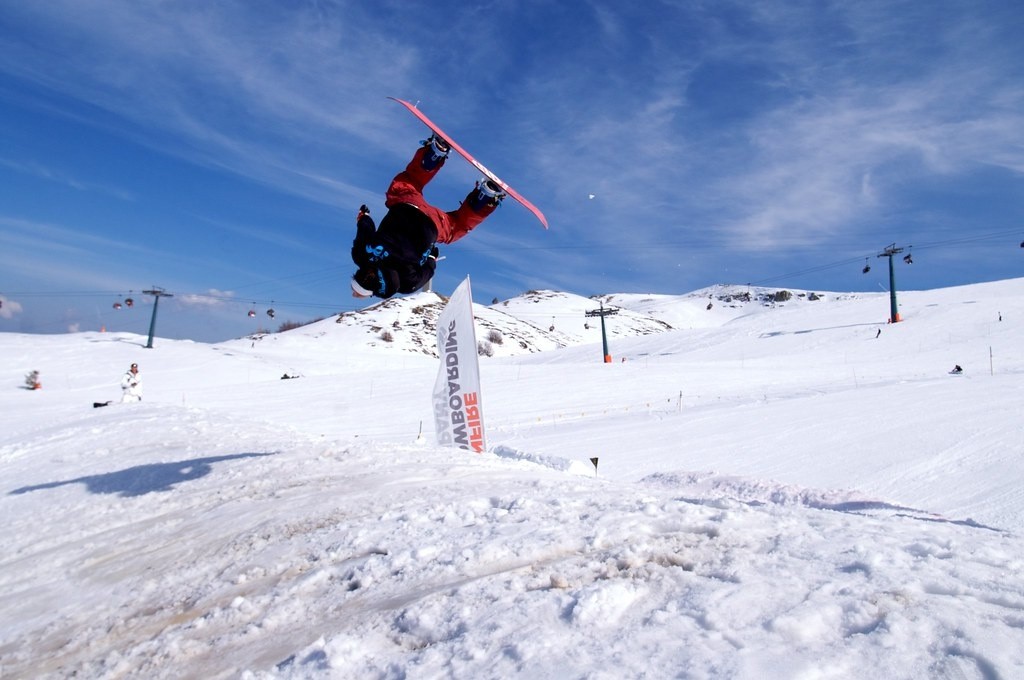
[351,270,382,299]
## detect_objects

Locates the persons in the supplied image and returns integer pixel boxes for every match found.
[351,135,507,299]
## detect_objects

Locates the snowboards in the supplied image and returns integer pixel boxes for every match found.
[386,96,549,230]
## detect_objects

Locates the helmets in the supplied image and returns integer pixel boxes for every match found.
[132,364,137,368]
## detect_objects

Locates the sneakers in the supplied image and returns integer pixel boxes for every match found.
[467,179,506,213]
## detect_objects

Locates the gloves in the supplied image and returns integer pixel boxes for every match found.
[429,245,439,258]
[359,205,370,213]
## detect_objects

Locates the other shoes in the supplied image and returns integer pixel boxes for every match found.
[420,138,450,173]
